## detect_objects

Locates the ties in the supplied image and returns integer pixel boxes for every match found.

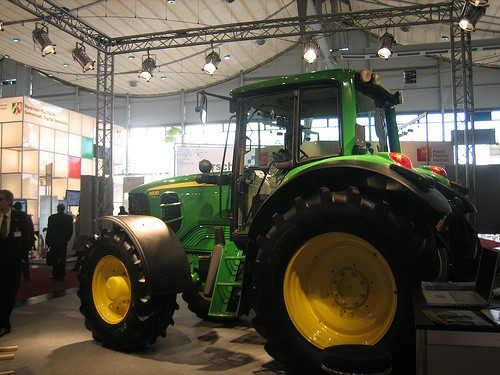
[1,214,8,242]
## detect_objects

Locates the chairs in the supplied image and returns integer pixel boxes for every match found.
[300,123,366,159]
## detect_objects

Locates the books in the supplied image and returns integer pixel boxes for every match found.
[421,282,489,307]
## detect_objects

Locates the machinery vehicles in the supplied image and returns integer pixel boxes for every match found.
[75,67,478,375]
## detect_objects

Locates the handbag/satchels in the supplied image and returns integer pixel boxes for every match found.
[47,245,54,266]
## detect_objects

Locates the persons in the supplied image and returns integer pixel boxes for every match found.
[45,204,73,281]
[72,207,89,272]
[118,206,128,215]
[0,190,28,337]
[10,202,34,295]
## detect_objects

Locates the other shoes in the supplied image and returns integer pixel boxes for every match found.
[59,277,65,280]
[0,326,11,336]
[50,275,59,279]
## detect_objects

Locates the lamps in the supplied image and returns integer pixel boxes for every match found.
[469,0,488,6]
[138,47,157,82]
[459,4,490,32]
[378,29,394,59]
[305,36,320,64]
[72,39,96,73]
[201,40,221,76]
[32,18,57,58]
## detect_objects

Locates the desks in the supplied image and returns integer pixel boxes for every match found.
[411,286,500,375]
